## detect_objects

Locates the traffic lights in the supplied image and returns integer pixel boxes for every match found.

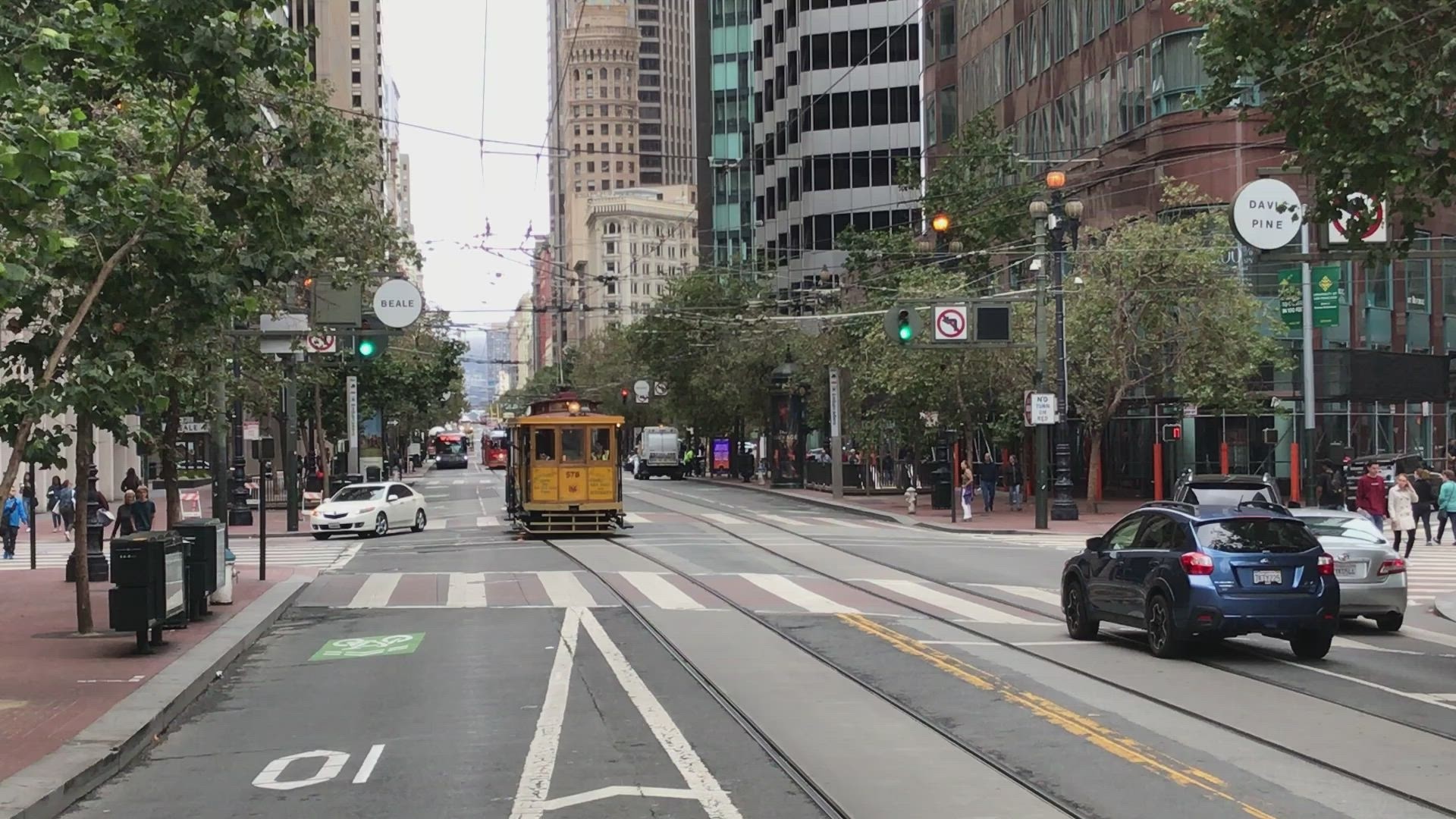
[1162,424,1181,442]
[1262,429,1278,444]
[344,314,388,360]
[883,304,923,343]
[934,217,948,264]
[973,303,1013,343]
[623,390,628,405]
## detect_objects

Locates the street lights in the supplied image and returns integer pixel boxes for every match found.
[1044,169,1084,522]
[1029,200,1050,532]
[1299,203,1344,508]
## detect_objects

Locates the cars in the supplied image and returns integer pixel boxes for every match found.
[176,459,210,470]
[459,411,487,425]
[309,481,428,540]
[1286,509,1408,632]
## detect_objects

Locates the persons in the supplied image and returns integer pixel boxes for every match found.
[1,486,26,559]
[961,460,973,522]
[97,467,155,551]
[1354,461,1386,534]
[841,446,860,464]
[592,440,610,461]
[1444,438,1456,476]
[46,476,76,542]
[1433,470,1456,546]
[978,453,999,512]
[684,446,695,477]
[296,447,330,476]
[1387,473,1419,559]
[540,452,551,461]
[1316,458,1345,510]
[1003,455,1022,511]
[18,473,31,534]
[1411,469,1433,547]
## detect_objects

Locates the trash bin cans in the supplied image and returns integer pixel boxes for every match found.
[210,547,235,603]
[107,531,187,633]
[170,517,225,616]
[931,469,953,509]
[330,474,363,496]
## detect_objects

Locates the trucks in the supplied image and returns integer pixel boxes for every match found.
[627,425,684,481]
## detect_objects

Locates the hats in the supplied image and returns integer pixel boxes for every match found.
[1320,459,1333,468]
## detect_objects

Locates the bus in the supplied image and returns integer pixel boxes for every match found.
[430,430,471,470]
[481,429,508,470]
[503,395,634,534]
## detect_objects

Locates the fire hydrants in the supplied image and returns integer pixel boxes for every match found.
[209,547,240,605]
[904,487,917,515]
[755,463,766,486]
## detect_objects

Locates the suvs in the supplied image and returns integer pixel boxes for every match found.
[1341,451,1446,519]
[1060,500,1341,659]
[1170,469,1282,507]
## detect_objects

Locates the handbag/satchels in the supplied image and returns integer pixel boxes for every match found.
[0,510,10,536]
[130,514,150,531]
[96,508,115,528]
[52,500,61,514]
[34,497,38,506]
[1437,509,1447,523]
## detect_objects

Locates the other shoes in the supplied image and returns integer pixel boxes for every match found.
[9,554,13,560]
[65,531,71,540]
[1453,542,1456,545]
[1433,538,1441,544]
[1404,558,1412,567]
[58,527,62,531]
[26,527,31,532]
[985,505,989,512]
[53,528,57,532]
[1427,540,1432,546]
[4,551,8,559]
[990,505,993,511]
